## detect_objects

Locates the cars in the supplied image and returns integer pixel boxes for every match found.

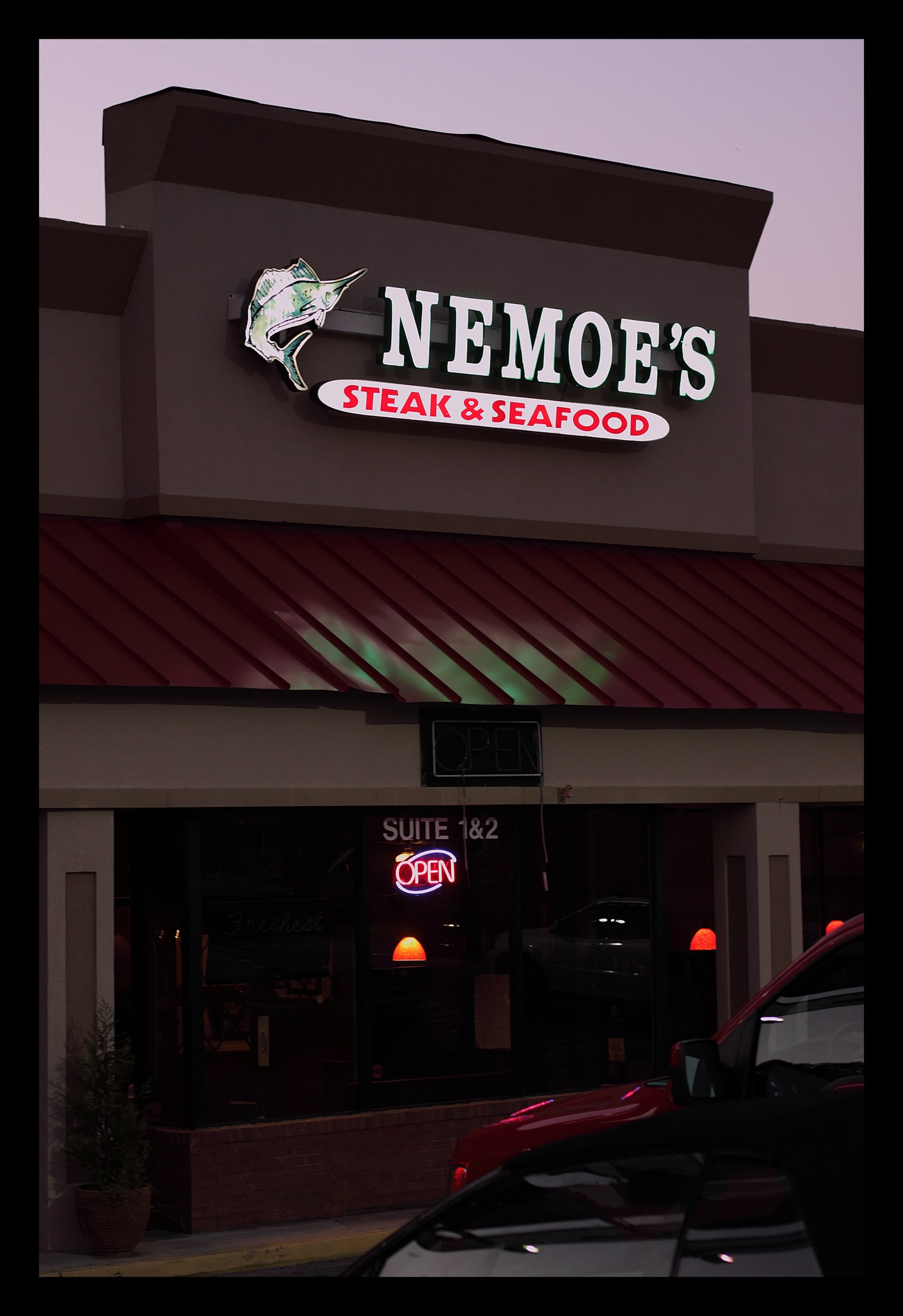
[448,913,862,1210]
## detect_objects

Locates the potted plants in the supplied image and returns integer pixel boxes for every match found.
[50,995,153,1257]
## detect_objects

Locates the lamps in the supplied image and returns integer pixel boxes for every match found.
[690,915,716,951]
[393,936,427,961]
